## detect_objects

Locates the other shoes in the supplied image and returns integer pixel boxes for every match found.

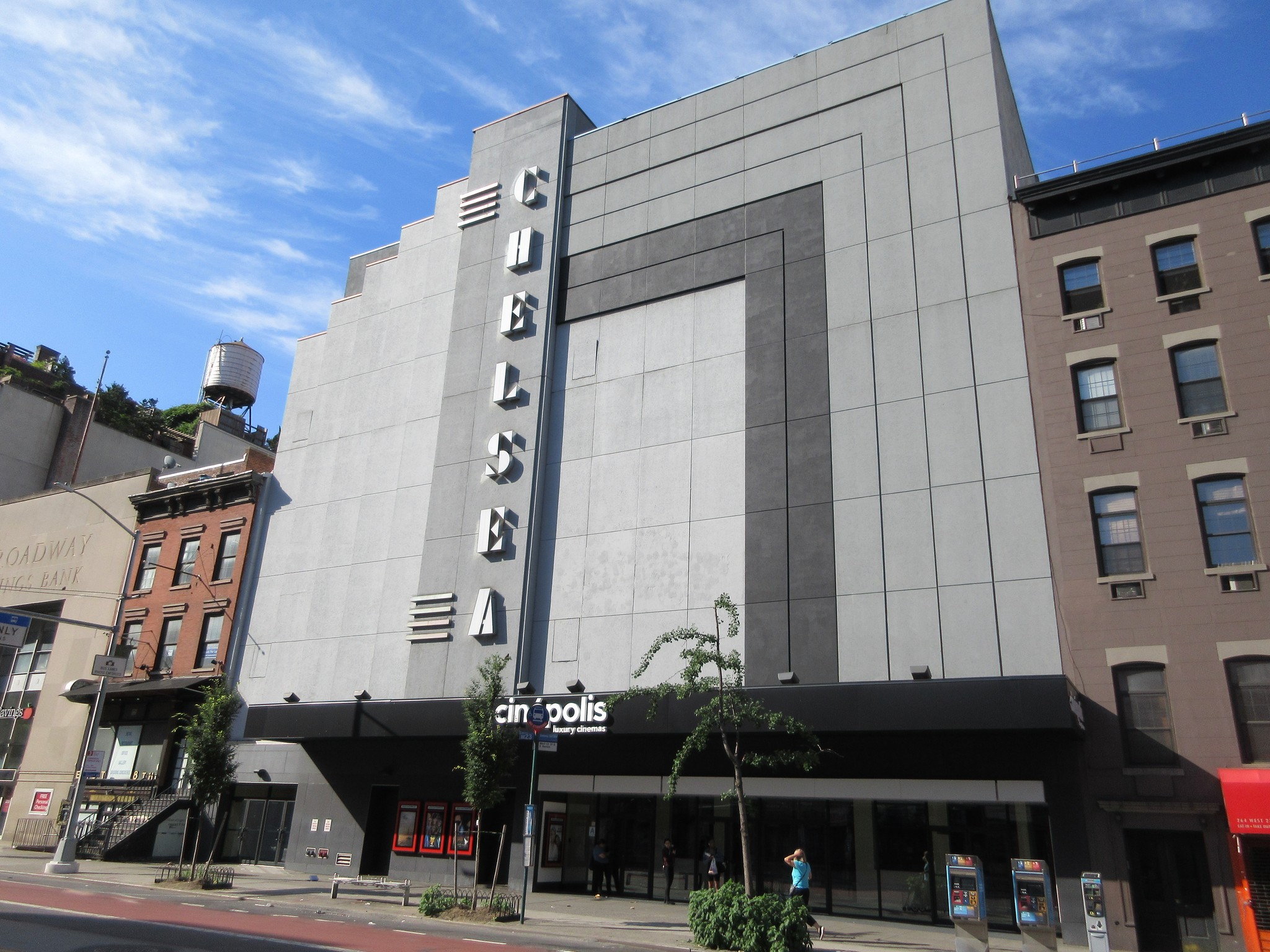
[818,925,824,941]
[664,899,676,905]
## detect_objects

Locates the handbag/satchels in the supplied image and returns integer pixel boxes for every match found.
[708,858,718,875]
[788,883,796,895]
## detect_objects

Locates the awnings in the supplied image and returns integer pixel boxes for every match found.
[58,677,218,703]
[242,675,1086,741]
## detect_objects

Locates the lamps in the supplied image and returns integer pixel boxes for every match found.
[517,682,535,695]
[777,672,800,684]
[910,665,932,680]
[139,664,149,671]
[354,690,371,700]
[283,692,300,703]
[211,659,222,665]
[565,679,585,693]
[253,769,266,774]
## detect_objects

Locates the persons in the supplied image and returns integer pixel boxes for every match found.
[548,827,562,862]
[922,851,937,922]
[593,840,620,898]
[784,848,825,940]
[661,838,675,904]
[703,839,726,892]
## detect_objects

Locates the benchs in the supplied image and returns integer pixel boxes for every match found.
[328,873,412,906]
